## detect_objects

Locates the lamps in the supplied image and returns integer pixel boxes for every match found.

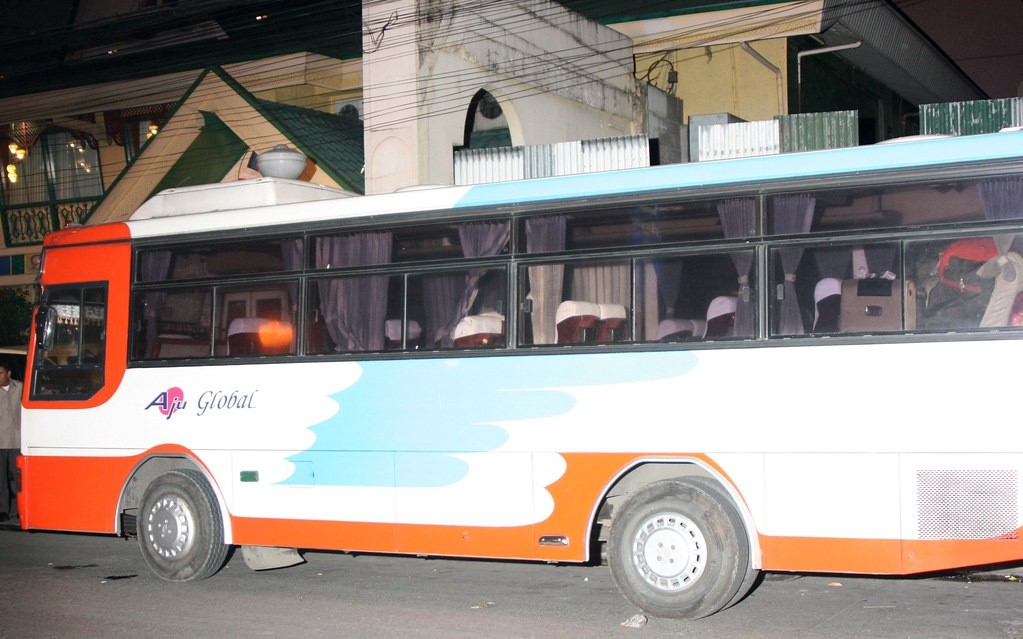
[254,143,308,180]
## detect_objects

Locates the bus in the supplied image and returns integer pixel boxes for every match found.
[15,130,1023,619]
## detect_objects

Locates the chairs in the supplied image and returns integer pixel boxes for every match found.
[554,299,601,345]
[384,319,423,350]
[452,316,503,348]
[702,296,737,337]
[979,252,1023,327]
[813,278,842,334]
[596,303,627,342]
[655,319,694,341]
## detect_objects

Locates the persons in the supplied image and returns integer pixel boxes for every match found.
[0,364,23,521]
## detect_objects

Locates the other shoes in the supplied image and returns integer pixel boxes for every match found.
[0,512,9,522]
